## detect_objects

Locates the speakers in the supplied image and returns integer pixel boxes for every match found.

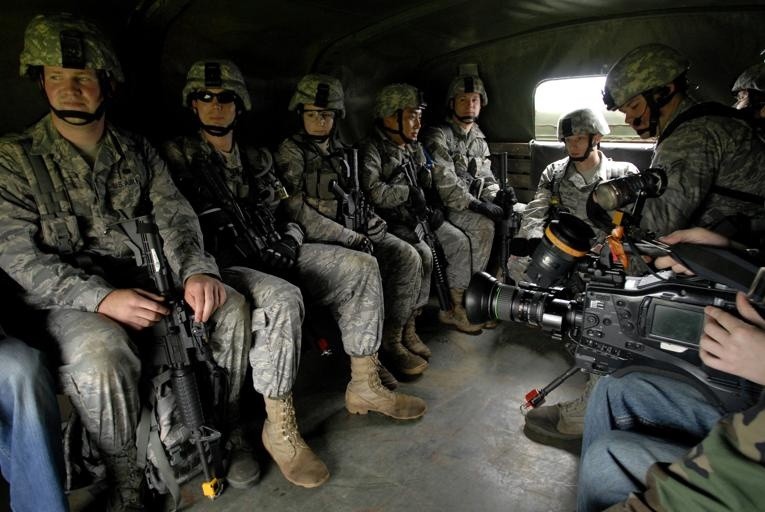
[260,393,331,488]
[522,367,607,447]
[375,338,430,374]
[404,313,433,358]
[107,455,147,511]
[218,405,265,489]
[439,286,499,332]
[343,350,427,420]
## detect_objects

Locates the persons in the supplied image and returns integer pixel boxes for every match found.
[166,58,427,490]
[507,108,640,286]
[525,43,765,442]
[273,73,429,389]
[0,327,70,512]
[358,84,482,359]
[575,225,765,512]
[0,11,262,512]
[731,63,765,120]
[422,72,527,335]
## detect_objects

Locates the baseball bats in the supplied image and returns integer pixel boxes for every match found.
[109,214,225,500]
[393,160,454,313]
[489,152,510,280]
[325,146,369,237]
[190,164,331,359]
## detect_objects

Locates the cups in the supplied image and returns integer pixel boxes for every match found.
[298,107,337,122]
[192,90,238,107]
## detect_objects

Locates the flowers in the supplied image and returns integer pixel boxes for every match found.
[633,88,660,126]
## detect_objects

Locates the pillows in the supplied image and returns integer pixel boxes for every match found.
[556,107,610,139]
[374,82,427,117]
[181,57,253,112]
[288,73,347,120]
[602,42,689,112]
[731,63,765,92]
[19,9,124,81]
[447,74,489,106]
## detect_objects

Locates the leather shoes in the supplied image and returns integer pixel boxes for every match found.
[428,208,444,231]
[256,234,302,278]
[493,186,517,209]
[367,211,388,244]
[468,196,504,219]
[338,232,373,255]
[405,185,427,210]
